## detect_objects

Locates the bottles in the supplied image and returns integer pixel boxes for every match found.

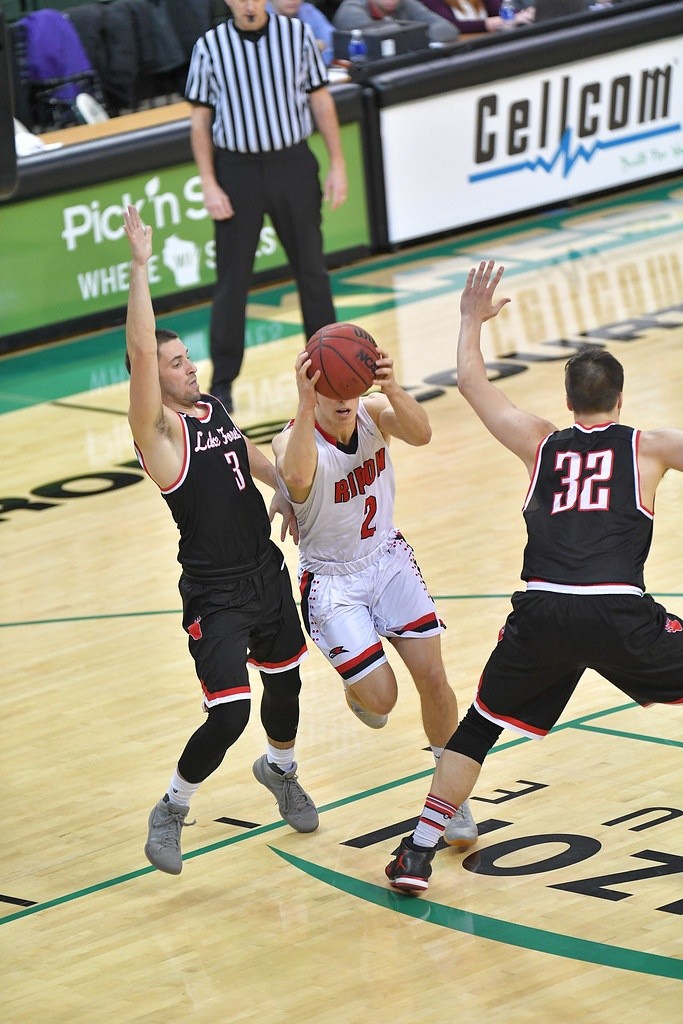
[348,29,368,64]
[500,0,515,26]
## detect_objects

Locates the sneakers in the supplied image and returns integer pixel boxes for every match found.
[444,799,478,845]
[385,836,438,891]
[252,754,319,833]
[345,688,389,729]
[144,798,197,875]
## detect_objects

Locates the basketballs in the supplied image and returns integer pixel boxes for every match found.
[302,321,382,402]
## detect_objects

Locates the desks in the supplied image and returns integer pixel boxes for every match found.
[37,102,194,148]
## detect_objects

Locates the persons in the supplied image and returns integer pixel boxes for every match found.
[271,346,478,847]
[384,259,683,891]
[121,206,321,874]
[331,0,460,42]
[185,0,347,417]
[446,0,536,30]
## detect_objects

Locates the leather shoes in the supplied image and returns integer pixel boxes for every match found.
[211,385,233,413]
